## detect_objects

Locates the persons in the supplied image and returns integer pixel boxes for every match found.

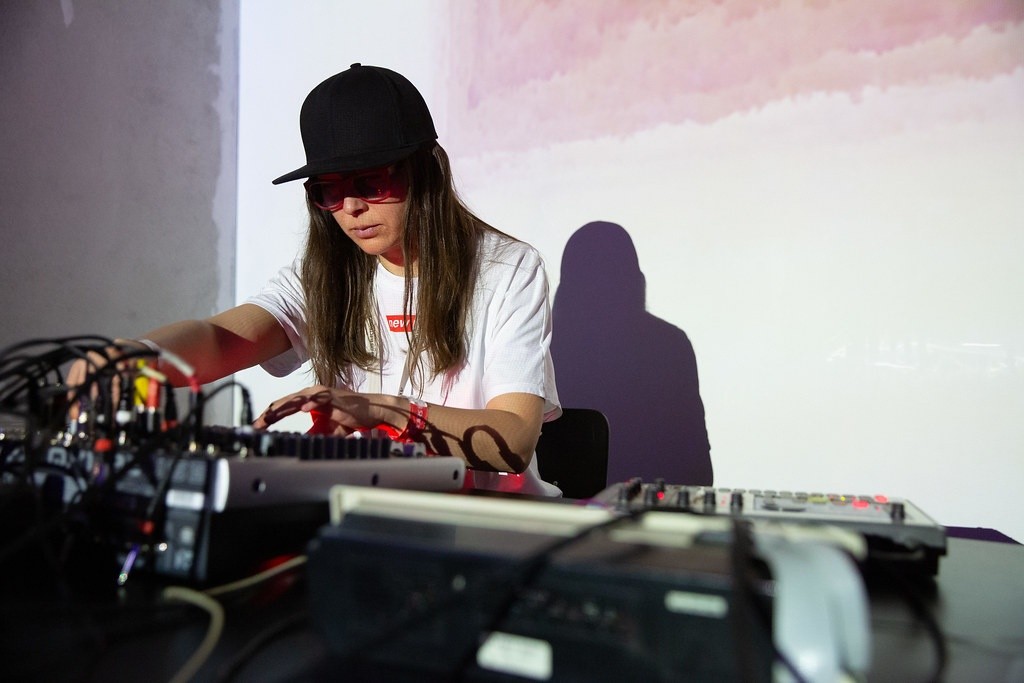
[67,64,562,484]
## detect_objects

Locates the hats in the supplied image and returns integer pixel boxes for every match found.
[272,63,438,185]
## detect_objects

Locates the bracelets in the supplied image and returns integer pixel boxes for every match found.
[400,396,428,443]
[138,339,160,351]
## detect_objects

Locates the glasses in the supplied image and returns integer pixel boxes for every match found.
[304,150,420,211]
